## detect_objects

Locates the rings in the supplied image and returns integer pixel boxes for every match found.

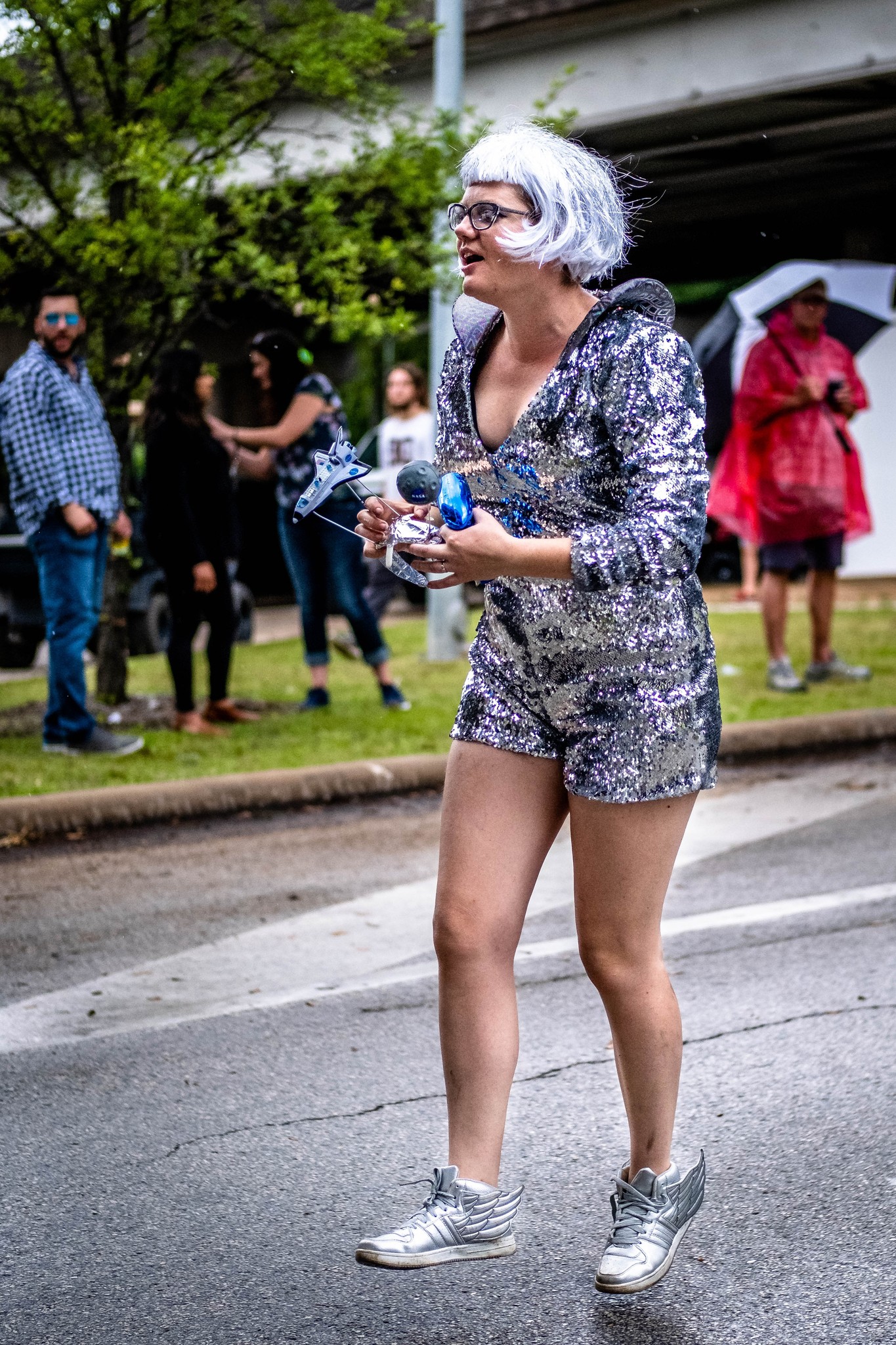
[441,560,446,574]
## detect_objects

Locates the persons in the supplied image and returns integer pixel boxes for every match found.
[0,279,147,762]
[705,278,876,694]
[350,126,723,1293]
[136,330,434,737]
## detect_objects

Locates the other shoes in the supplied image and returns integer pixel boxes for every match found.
[332,636,360,659]
[767,658,807,693]
[805,651,874,682]
[300,688,328,710]
[380,682,410,711]
[204,699,260,722]
[42,727,145,758]
[175,711,225,733]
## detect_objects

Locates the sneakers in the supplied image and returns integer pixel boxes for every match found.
[355,1165,525,1269]
[594,1149,706,1294]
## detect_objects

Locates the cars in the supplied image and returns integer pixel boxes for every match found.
[0,490,257,673]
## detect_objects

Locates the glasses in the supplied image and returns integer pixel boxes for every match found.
[46,312,79,326]
[800,294,825,305]
[448,202,537,230]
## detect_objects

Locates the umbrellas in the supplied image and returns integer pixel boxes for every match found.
[692,259,896,453]
